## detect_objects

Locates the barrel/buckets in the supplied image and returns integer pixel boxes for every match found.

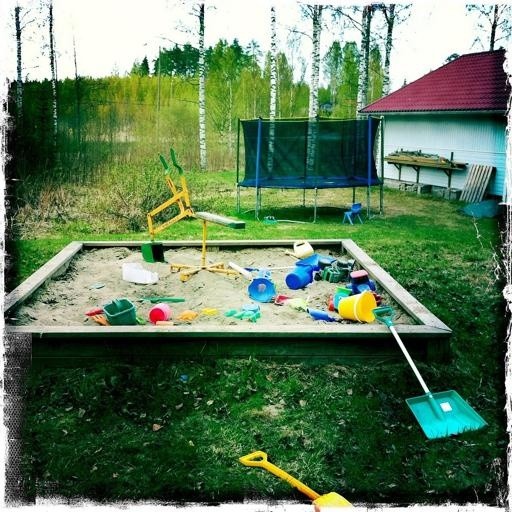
[339,289,377,322]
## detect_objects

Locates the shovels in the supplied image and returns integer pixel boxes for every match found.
[372,306,489,441]
[239,451,354,506]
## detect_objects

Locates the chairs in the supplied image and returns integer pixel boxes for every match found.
[342,202,364,226]
